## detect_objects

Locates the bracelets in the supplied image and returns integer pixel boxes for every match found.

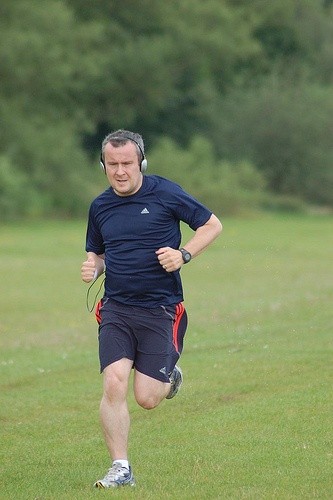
[103,261,106,271]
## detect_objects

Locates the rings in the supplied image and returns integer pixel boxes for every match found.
[164,266,167,269]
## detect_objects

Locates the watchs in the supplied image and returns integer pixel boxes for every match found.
[179,248,191,264]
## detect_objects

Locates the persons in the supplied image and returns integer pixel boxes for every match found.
[81,130,222,488]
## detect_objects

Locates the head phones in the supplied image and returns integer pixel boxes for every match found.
[98,135,147,173]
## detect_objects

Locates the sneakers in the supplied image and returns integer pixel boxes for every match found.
[165,365,183,399]
[92,462,136,488]
